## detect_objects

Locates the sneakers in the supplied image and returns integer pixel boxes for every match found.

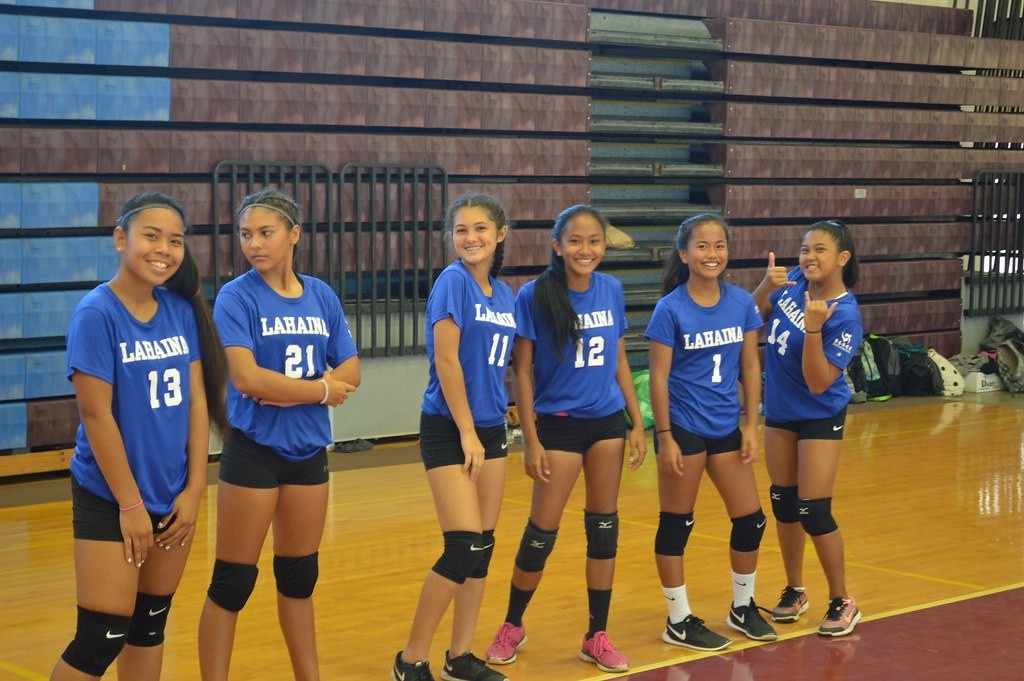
[728,596,779,640]
[441,650,509,681]
[771,585,809,622]
[485,623,528,665]
[819,596,862,637]
[391,651,435,681]
[662,614,733,651]
[579,631,631,673]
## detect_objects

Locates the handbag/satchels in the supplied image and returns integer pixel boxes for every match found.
[927,349,965,397]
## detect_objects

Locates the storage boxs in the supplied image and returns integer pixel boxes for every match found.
[962,371,1006,393]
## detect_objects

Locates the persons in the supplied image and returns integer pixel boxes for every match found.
[390,192,516,681]
[486,204,648,673]
[644,212,778,652]
[198,187,361,681]
[750,219,863,637]
[49,191,229,681]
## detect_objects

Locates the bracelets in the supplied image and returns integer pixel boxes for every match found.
[318,380,329,404]
[805,331,820,334]
[119,499,143,510]
[656,429,672,435]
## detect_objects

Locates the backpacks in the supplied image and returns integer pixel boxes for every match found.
[894,335,945,396]
[858,334,900,402]
[996,338,1024,396]
[979,315,1024,351]
[841,353,870,403]
